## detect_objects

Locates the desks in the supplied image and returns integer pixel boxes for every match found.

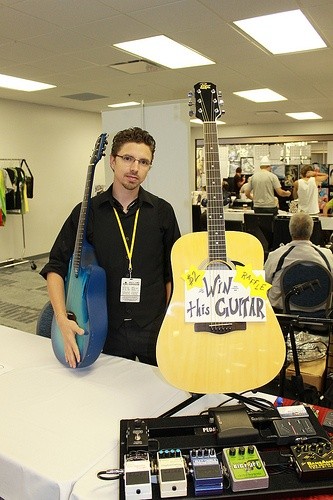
[0,322,333,500]
[223,208,333,232]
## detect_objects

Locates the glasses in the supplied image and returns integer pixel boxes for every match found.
[115,154,152,167]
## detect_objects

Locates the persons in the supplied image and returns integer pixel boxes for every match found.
[38,128,181,366]
[264,212,333,316]
[222,158,333,217]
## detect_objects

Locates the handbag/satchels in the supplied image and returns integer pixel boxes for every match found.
[21,159,34,198]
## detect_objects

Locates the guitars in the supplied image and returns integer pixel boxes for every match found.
[155,81,287,394]
[50,132,110,368]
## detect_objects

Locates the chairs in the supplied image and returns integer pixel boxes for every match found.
[193,205,333,376]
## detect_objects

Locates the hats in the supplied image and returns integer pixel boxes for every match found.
[260,156,271,165]
